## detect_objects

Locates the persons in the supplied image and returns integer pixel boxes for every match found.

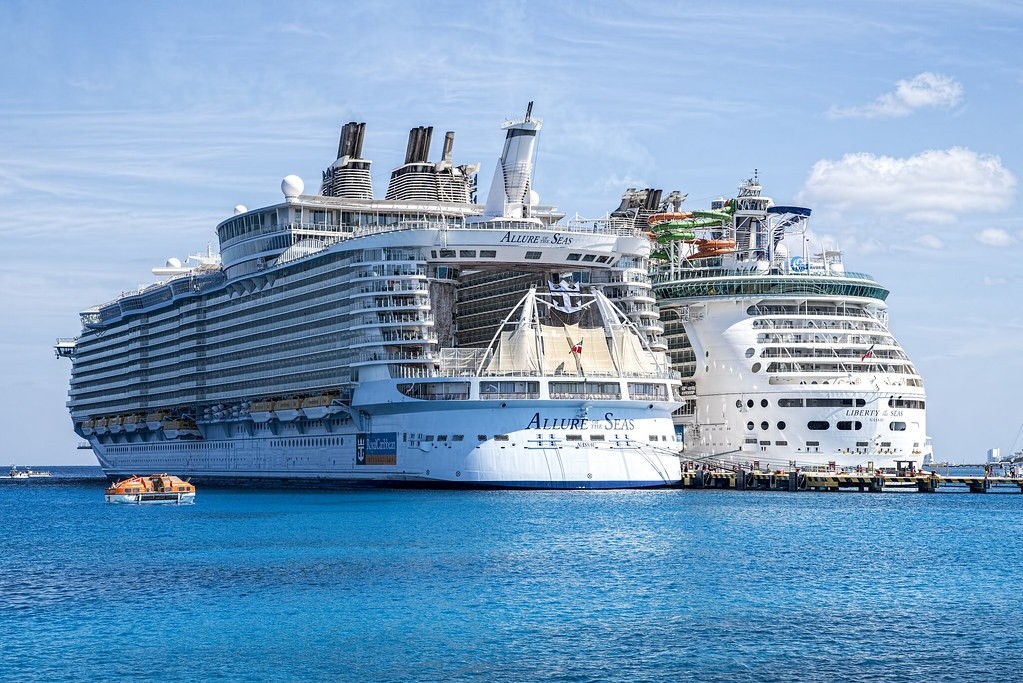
[984,465,1020,478]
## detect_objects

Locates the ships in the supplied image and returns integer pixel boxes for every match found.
[51,98,690,492]
[606,166,929,494]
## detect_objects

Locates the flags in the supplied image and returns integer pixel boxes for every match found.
[862,345,874,362]
[569,341,581,354]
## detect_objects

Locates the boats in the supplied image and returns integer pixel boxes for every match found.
[122,414,147,433]
[94,417,110,435]
[146,412,170,431]
[274,395,306,422]
[81,418,97,437]
[247,399,277,423]
[103,473,197,506]
[300,394,341,421]
[0,464,54,480]
[108,415,126,435]
[162,418,202,440]
